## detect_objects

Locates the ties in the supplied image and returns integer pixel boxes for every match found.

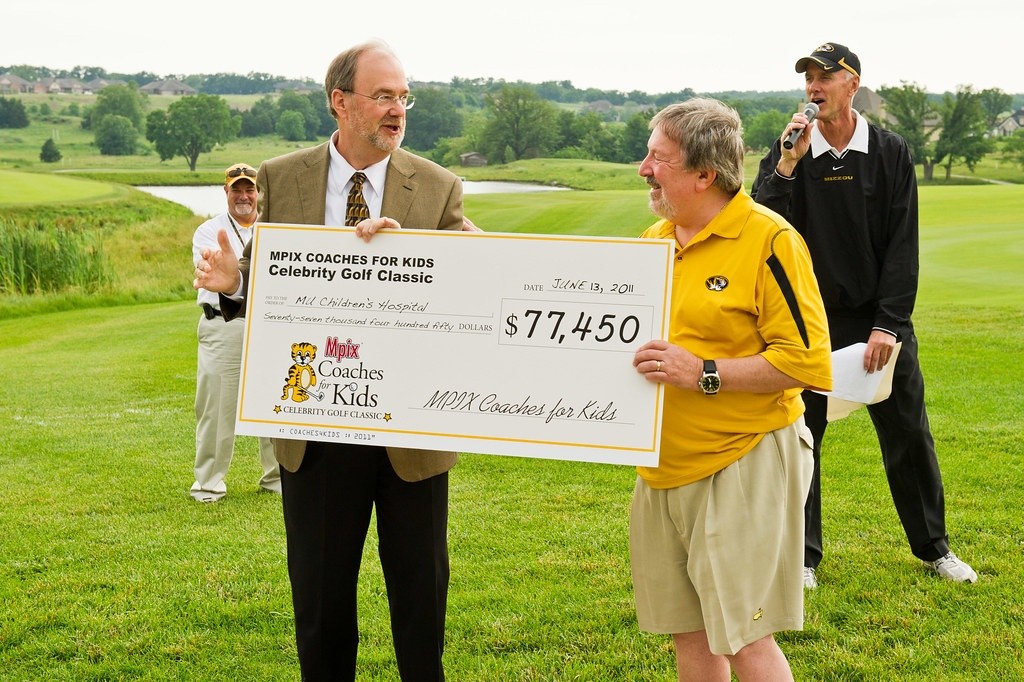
[344,171,371,227]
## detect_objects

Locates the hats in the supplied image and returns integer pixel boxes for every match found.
[224,162,258,185]
[794,42,862,81]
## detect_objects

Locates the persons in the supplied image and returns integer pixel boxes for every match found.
[750,42,979,591]
[189,163,282,503]
[461,98,834,682]
[193,40,464,682]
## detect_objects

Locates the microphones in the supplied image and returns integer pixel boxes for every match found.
[783,102,819,149]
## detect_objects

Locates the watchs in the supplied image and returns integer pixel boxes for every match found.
[698,359,721,395]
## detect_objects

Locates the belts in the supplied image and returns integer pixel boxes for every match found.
[213,309,245,318]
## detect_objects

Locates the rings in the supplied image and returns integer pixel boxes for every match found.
[657,360,662,371]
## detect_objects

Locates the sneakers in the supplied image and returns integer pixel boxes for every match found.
[922,551,978,587]
[802,566,820,590]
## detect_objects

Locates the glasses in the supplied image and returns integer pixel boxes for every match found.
[228,167,258,178]
[340,87,416,112]
